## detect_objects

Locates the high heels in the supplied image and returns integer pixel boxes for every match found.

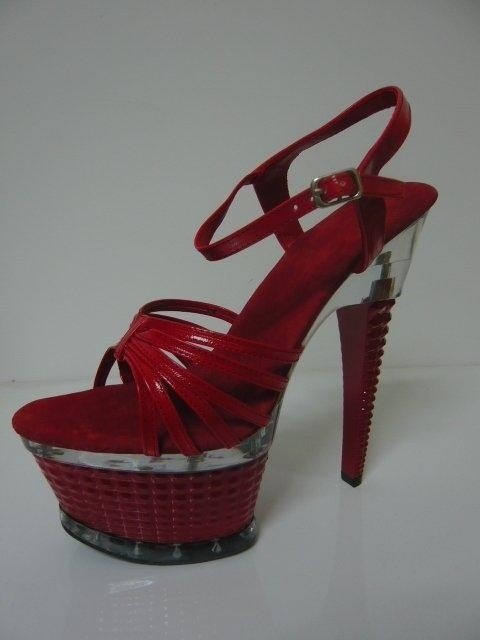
[11,86,439,566]
